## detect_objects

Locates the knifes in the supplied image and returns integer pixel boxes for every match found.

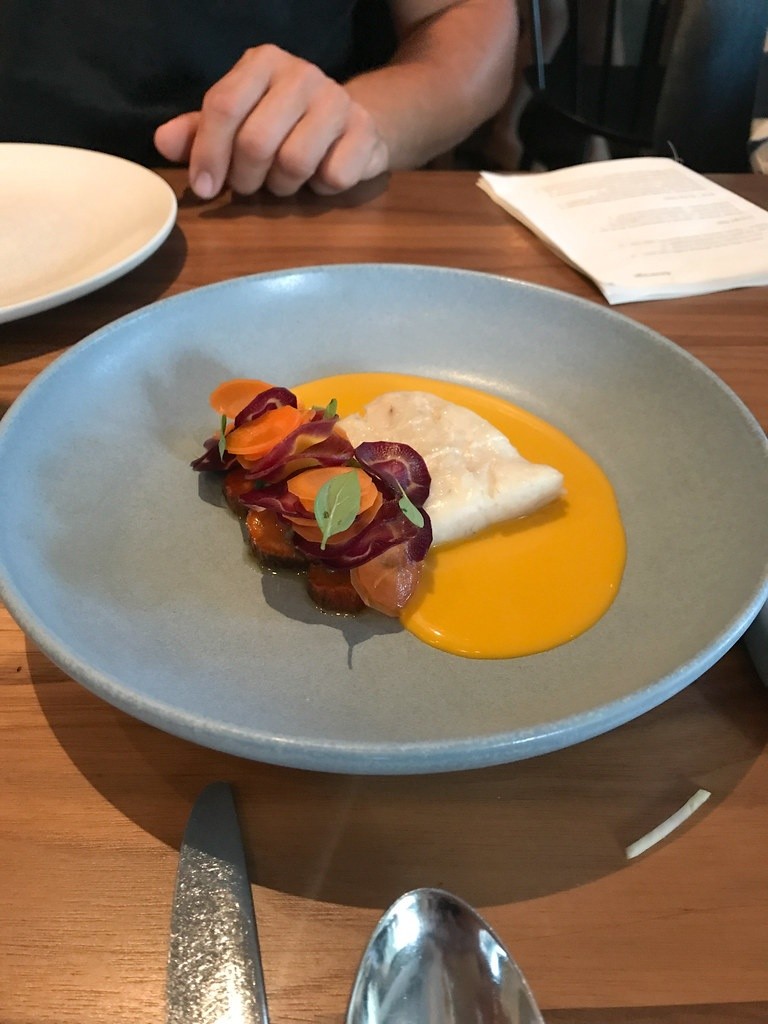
[168,781,269,1024]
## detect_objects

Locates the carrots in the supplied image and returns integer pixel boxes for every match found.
[189,379,433,616]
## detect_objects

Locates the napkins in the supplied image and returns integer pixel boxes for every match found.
[476,157,768,305]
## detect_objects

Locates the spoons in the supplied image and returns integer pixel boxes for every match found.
[346,886,544,1024]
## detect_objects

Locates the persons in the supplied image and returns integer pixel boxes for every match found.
[0,1,518,198]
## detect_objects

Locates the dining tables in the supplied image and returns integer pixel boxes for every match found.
[0,167,768,1023]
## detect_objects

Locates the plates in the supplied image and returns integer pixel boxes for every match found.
[0,263,768,771]
[0,142,177,322]
